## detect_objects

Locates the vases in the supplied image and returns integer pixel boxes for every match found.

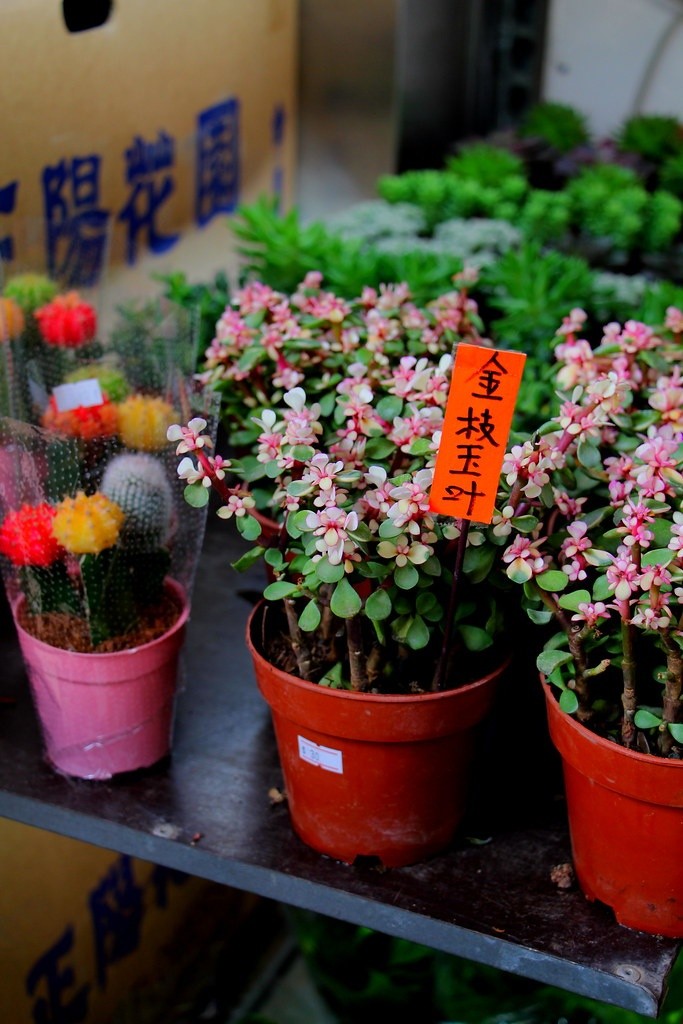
[245,599,510,871]
[11,575,192,781]
[533,664,683,943]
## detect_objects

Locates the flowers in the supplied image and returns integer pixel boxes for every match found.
[0,270,183,629]
[165,262,505,693]
[493,305,683,759]
